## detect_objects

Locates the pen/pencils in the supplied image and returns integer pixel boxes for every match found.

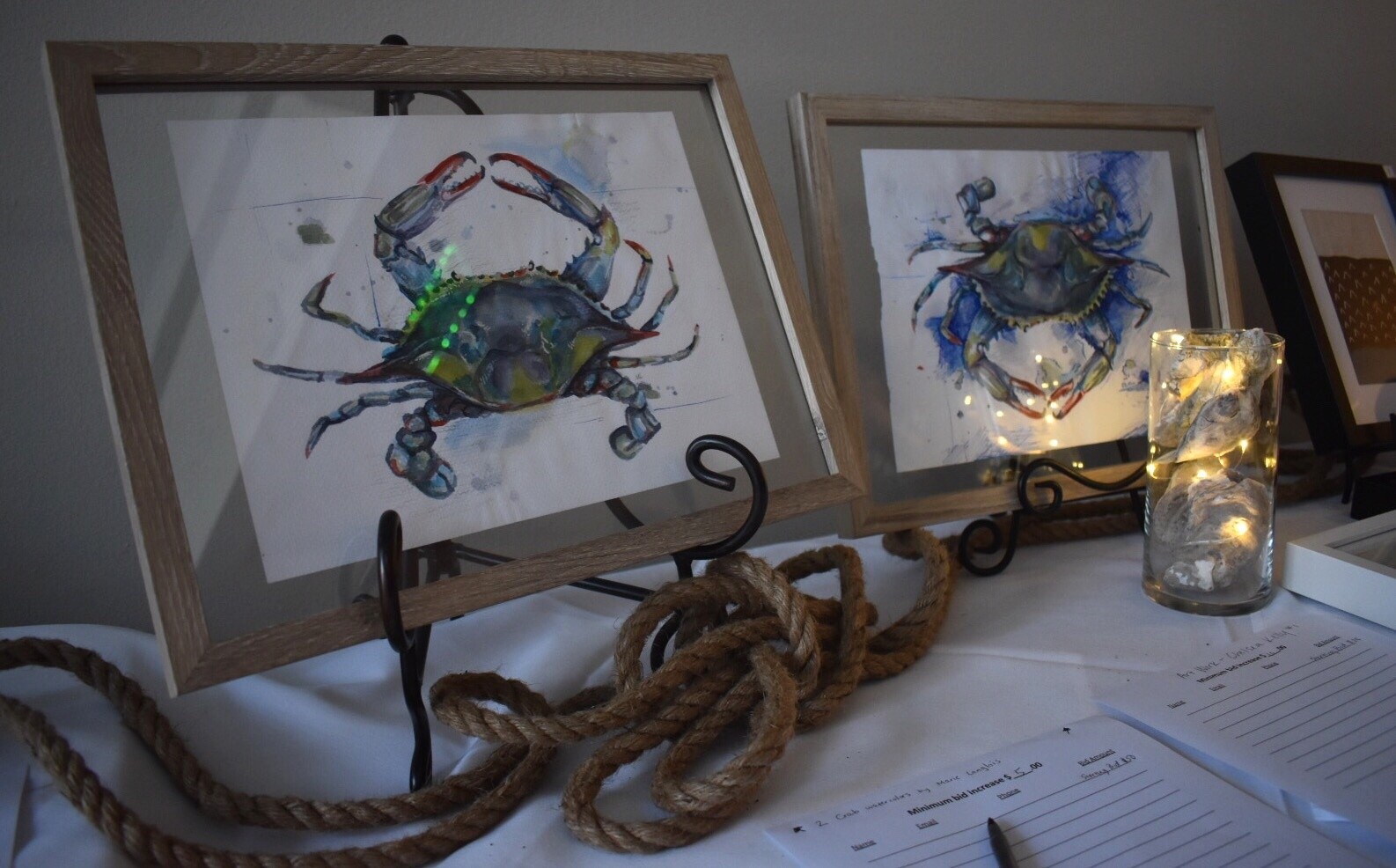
[987,817,1017,868]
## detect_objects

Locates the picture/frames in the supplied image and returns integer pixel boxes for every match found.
[41,40,837,698]
[1227,154,1396,516]
[788,90,1237,541]
[1283,511,1395,631]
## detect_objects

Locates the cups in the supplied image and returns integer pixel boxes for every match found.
[1140,327,1291,614]
